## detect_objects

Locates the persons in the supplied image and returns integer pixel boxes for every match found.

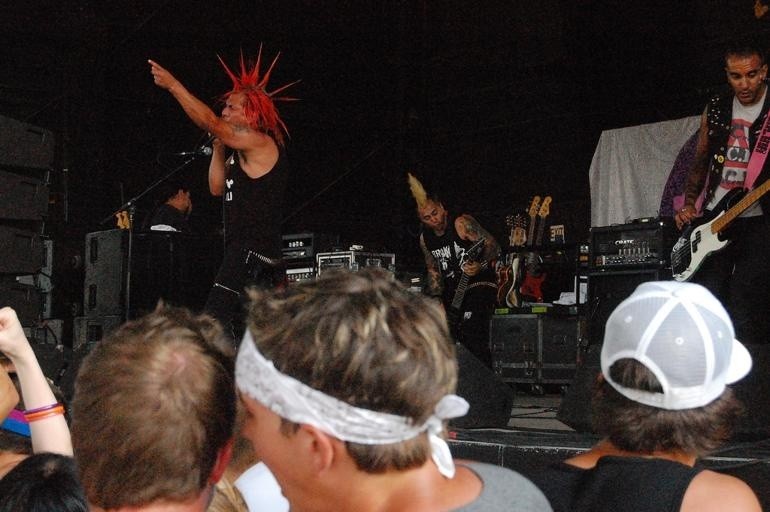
[148,58,302,268]
[140,177,195,233]
[551,280,762,512]
[675,46,769,345]
[0,264,553,512]
[417,193,500,370]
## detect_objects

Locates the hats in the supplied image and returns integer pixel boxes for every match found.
[601,281,754,409]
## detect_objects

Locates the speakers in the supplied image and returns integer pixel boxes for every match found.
[0,166,52,224]
[73,315,123,351]
[0,227,49,276]
[584,267,674,369]
[0,280,45,325]
[25,335,68,381]
[84,227,129,312]
[0,116,56,170]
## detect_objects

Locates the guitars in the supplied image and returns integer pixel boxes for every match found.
[444,239,485,344]
[672,177,770,282]
[497,196,553,308]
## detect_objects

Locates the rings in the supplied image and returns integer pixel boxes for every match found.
[681,208,687,212]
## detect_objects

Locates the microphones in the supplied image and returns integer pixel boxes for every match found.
[179,147,213,157]
[462,237,488,260]
